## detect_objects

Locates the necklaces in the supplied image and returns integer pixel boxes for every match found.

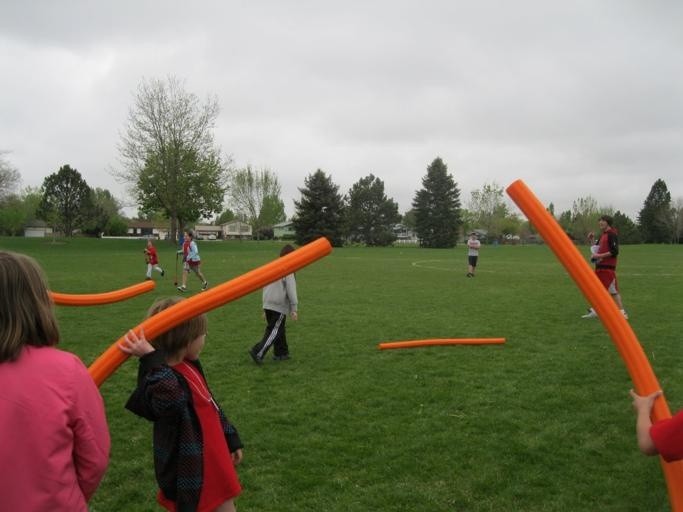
[170,362,220,411]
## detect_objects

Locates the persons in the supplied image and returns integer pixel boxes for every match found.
[466,233,481,278]
[249,245,298,367]
[630,387,683,463]
[580,214,629,319]
[177,230,208,293]
[118,296,243,512]
[1,250,112,511]
[144,239,164,280]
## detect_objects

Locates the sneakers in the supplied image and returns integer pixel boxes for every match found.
[202,282,207,290]
[582,308,597,318]
[177,286,185,292]
